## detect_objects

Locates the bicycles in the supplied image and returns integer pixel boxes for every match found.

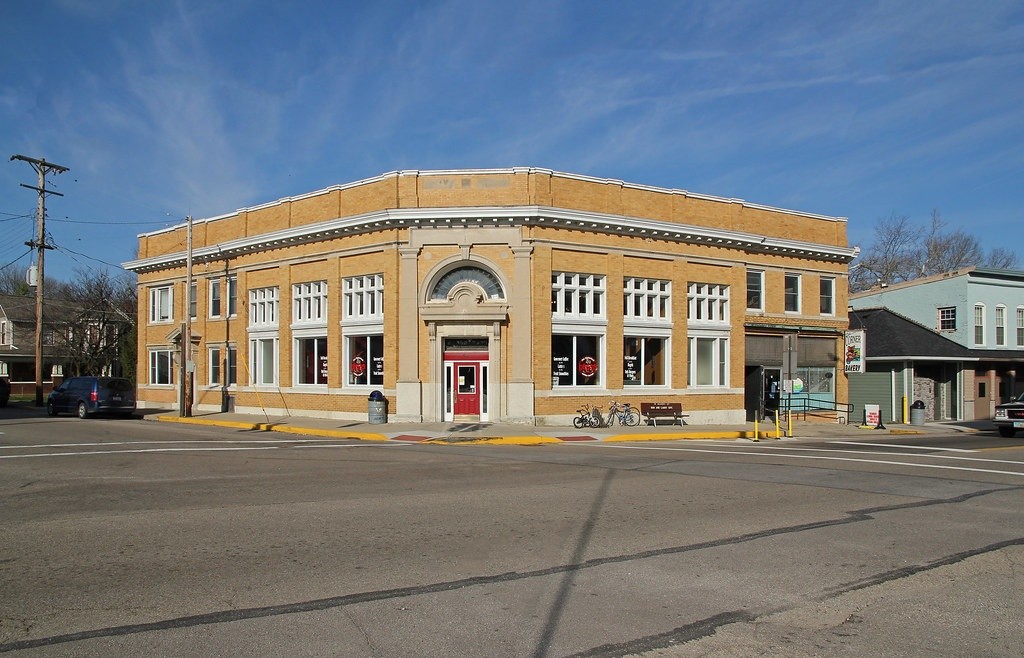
[605,401,640,427]
[572,404,600,428]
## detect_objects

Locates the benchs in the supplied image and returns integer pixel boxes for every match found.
[641,402,689,427]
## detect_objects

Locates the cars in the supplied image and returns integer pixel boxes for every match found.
[992,391,1024,439]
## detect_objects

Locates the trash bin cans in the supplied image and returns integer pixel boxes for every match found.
[910,400,926,424]
[367,390,387,426]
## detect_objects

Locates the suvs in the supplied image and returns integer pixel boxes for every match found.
[45,375,137,419]
